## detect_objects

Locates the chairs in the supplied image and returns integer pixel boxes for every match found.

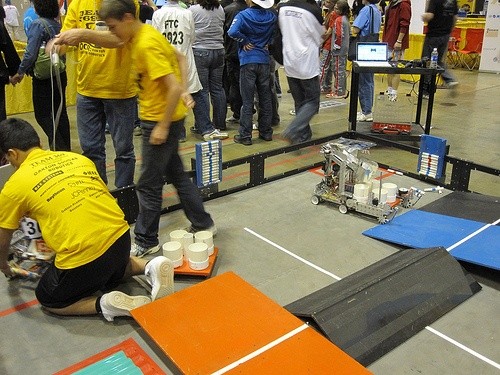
[447,27,484,70]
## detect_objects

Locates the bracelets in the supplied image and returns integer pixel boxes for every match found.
[397,40,402,43]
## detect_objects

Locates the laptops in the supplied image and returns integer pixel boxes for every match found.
[355,42,392,68]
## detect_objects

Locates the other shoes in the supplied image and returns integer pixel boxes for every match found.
[253,124,259,129]
[287,89,291,93]
[190,127,200,134]
[290,110,296,115]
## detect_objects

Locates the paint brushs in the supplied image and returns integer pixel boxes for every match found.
[411,187,425,195]
[201,140,220,186]
[424,187,444,192]
[418,152,439,179]
[388,169,403,176]
[437,186,442,195]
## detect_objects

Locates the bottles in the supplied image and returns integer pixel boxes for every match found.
[40,41,46,49]
[430,48,438,69]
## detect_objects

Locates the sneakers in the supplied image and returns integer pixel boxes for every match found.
[100,291,152,322]
[357,111,373,121]
[203,129,228,141]
[416,92,429,99]
[226,117,239,122]
[130,242,160,257]
[320,87,332,93]
[259,134,272,140]
[378,91,397,101]
[182,224,217,238]
[277,94,281,98]
[447,82,459,87]
[145,256,175,301]
[326,93,347,98]
[234,135,251,144]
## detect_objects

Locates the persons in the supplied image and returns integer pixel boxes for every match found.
[151,0,393,131]
[269,0,326,156]
[0,117,174,322]
[418,0,459,99]
[225,0,277,146]
[23,0,40,38]
[222,0,249,123]
[152,0,203,143]
[46,0,138,187]
[458,4,470,18]
[383,0,411,102]
[3,0,21,41]
[9,0,71,152]
[97,0,217,258]
[138,0,154,23]
[325,2,351,99]
[0,1,22,166]
[186,0,227,134]
[351,0,381,122]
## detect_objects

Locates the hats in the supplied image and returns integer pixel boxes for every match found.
[252,0,274,9]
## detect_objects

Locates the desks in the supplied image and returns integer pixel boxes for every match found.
[349,60,445,141]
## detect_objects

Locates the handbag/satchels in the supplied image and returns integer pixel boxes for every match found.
[268,22,283,66]
[34,46,66,79]
[348,33,379,61]
